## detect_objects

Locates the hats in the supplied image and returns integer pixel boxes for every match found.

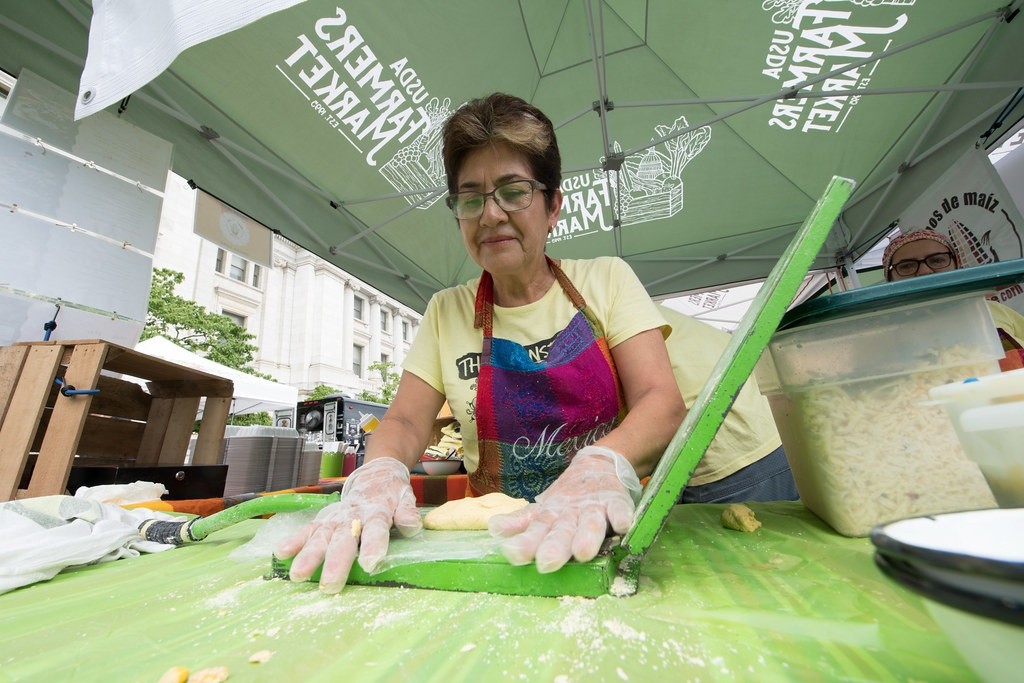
[882,229,961,281]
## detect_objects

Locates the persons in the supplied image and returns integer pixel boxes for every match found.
[653,305,799,504]
[883,229,1024,372]
[280,91,688,594]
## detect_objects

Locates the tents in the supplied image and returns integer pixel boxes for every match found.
[101,335,298,429]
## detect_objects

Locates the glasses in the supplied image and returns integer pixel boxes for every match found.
[888,251,955,276]
[446,179,548,220]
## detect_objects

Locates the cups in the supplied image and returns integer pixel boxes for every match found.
[320,451,345,477]
[343,452,356,477]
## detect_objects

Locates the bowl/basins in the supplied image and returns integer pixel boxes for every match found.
[410,461,426,473]
[870,506,1024,683]
[422,460,462,474]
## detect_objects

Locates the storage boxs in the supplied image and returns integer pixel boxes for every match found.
[753,291,1007,538]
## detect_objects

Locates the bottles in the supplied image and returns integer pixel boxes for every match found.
[357,410,381,432]
